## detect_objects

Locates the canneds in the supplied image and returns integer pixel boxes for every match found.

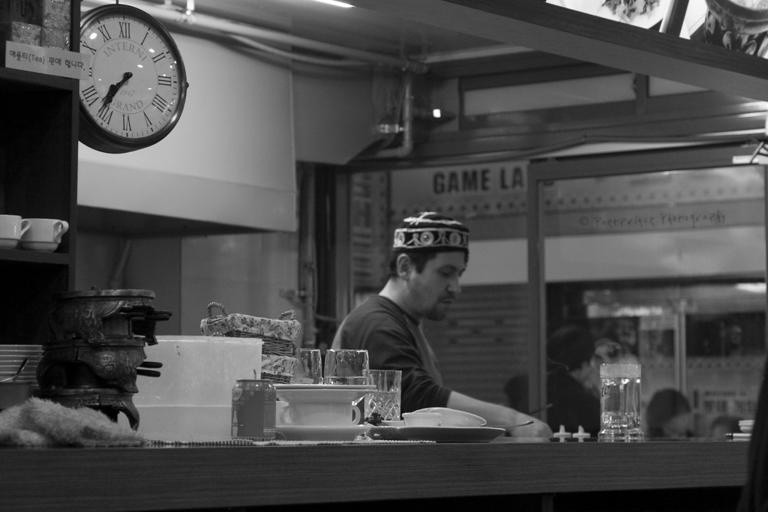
[231,379,276,441]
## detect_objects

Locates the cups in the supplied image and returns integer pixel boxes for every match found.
[0,214,31,252]
[21,218,69,252]
[599,362,647,442]
[291,348,403,426]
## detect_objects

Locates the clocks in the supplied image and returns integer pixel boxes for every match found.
[75,4,189,154]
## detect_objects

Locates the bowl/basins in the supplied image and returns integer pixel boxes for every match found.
[737,420,755,431]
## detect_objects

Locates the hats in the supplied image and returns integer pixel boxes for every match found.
[390,206,474,253]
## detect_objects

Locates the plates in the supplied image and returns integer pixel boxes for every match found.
[268,381,507,442]
[0,344,47,391]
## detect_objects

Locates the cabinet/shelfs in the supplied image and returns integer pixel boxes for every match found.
[1,2,80,409]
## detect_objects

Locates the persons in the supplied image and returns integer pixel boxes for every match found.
[709,415,745,439]
[725,325,758,356]
[611,317,638,357]
[331,210,555,441]
[503,329,602,442]
[646,387,695,438]
